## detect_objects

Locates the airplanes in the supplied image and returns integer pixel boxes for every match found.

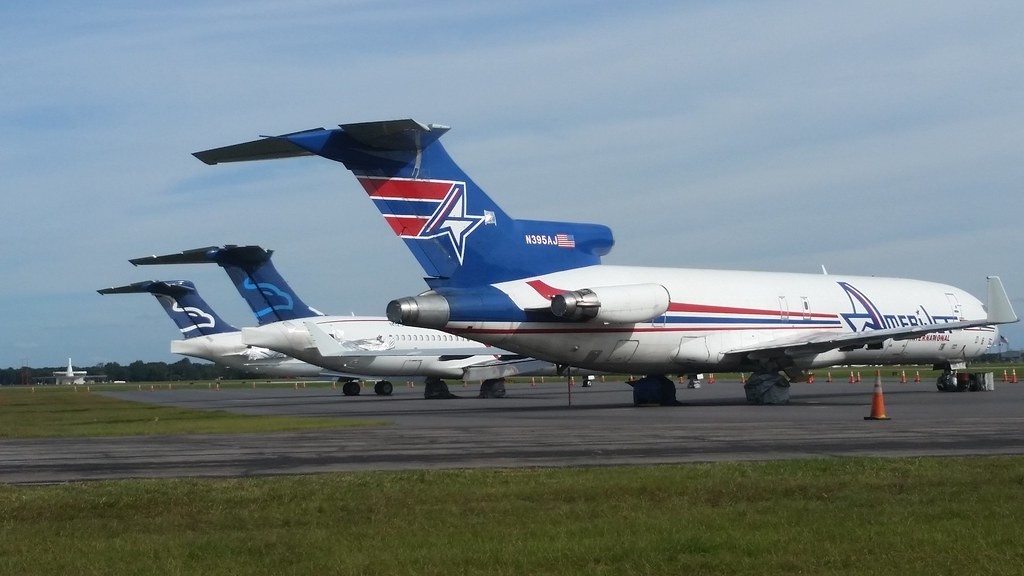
[128,244,635,400]
[97,280,428,397]
[191,118,1022,409]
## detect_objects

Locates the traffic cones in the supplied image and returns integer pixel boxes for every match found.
[531,375,536,386]
[1002,369,1009,382]
[679,376,685,384]
[1010,368,1019,383]
[900,369,907,383]
[569,376,576,385]
[857,370,863,382]
[808,371,814,384]
[848,370,856,383]
[913,370,922,383]
[825,372,833,382]
[864,369,892,420]
[628,375,635,381]
[740,372,746,384]
[540,376,545,384]
[139,380,418,393]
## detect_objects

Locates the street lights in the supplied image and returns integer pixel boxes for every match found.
[708,373,716,385]
